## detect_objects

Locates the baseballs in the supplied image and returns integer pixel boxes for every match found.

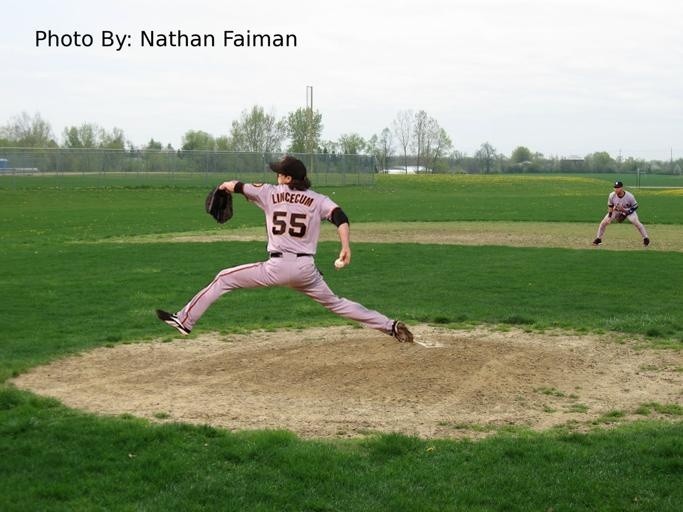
[334,258,345,269]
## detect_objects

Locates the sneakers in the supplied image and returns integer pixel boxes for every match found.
[594,239,601,244]
[156,309,190,336]
[393,321,413,343]
[643,238,649,245]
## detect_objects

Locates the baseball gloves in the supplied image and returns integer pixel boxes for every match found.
[614,211,627,223]
[205,185,234,224]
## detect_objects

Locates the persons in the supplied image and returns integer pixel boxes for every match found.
[591,182,651,245]
[154,156,416,343]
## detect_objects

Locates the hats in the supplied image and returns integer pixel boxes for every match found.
[269,157,306,178]
[614,182,623,188]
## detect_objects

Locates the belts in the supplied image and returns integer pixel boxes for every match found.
[271,251,310,257]
